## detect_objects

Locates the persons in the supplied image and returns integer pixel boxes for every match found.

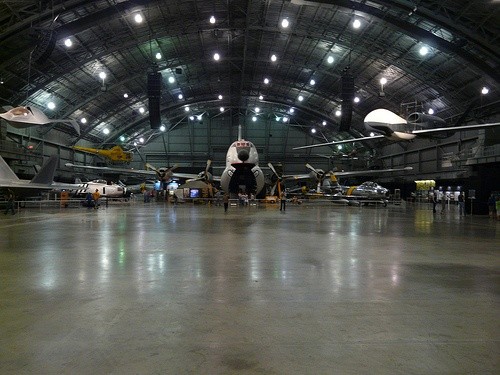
[432,193,437,213]
[85,192,92,209]
[239,193,255,207]
[224,192,229,212]
[173,194,178,207]
[280,189,286,211]
[4,187,16,215]
[93,189,100,208]
[458,191,465,208]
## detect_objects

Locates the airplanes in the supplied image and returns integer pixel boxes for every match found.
[0,106,414,207]
[292,108,500,150]
